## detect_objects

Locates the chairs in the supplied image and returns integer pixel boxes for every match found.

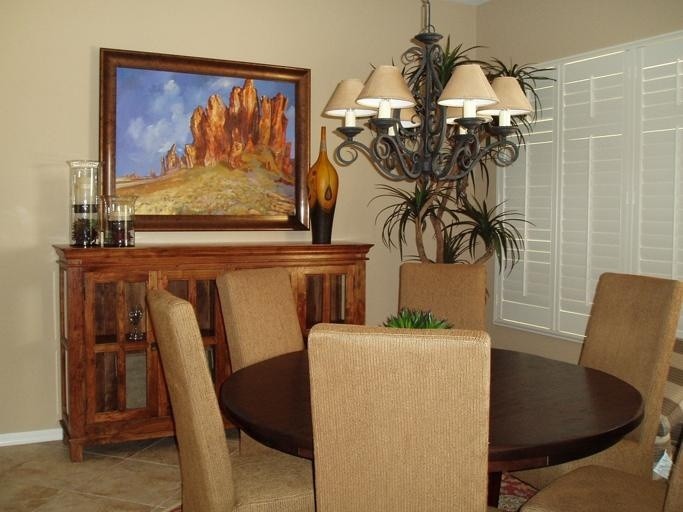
[144,287,316,512]
[509,272,683,491]
[215,266,306,457]
[397,262,487,331]
[516,437,683,512]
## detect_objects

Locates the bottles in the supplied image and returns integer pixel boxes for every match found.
[67,159,137,248]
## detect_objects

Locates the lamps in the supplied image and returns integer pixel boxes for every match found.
[321,0,535,181]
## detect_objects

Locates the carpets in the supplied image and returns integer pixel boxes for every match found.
[166,473,538,512]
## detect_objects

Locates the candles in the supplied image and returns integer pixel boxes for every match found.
[73,174,96,204]
[108,204,131,222]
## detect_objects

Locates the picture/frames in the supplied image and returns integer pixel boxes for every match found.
[99,47,311,232]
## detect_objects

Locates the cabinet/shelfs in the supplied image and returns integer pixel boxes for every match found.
[54,245,229,462]
[224,244,373,379]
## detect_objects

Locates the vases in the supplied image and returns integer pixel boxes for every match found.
[305,126,339,244]
